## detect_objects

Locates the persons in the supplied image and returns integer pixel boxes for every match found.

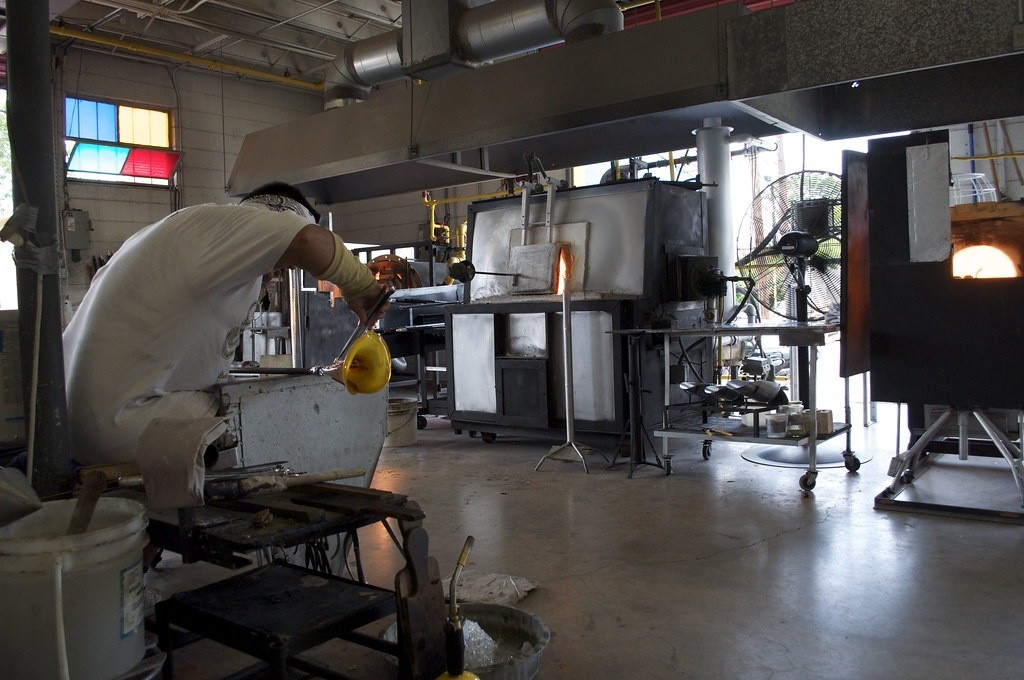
[65,180,391,465]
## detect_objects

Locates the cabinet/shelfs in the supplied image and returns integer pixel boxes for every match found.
[147,483,452,680]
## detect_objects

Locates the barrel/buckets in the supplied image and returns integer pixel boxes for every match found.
[378,602,549,680]
[765,414,787,437]
[382,398,418,448]
[0,497,151,680]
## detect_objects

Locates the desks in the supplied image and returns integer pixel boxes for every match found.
[606,320,850,472]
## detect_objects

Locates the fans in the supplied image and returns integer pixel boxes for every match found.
[735,170,875,469]
